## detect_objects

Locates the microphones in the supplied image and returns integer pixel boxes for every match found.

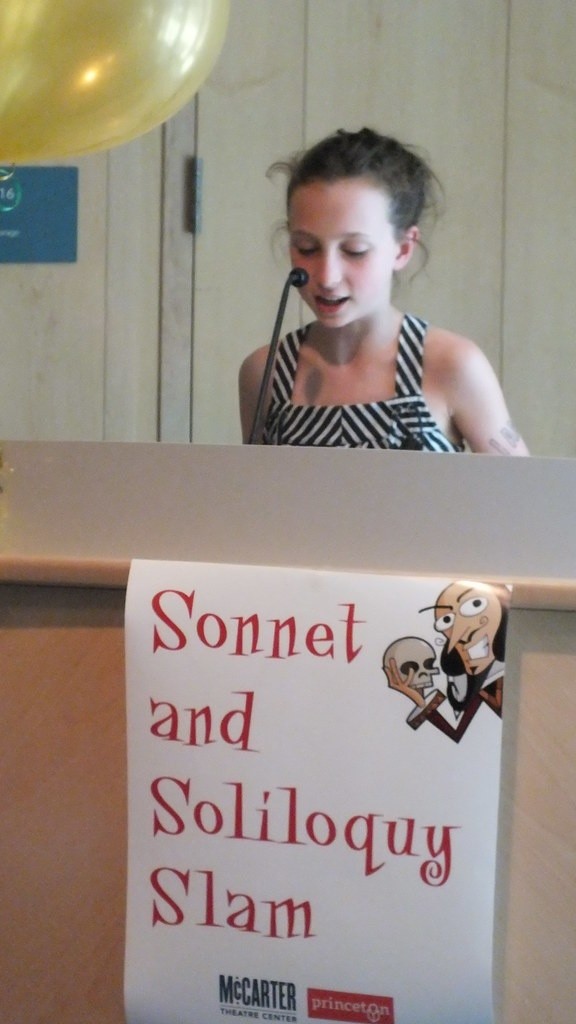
[248,267,309,444]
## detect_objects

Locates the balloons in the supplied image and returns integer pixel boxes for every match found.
[0,0,229,163]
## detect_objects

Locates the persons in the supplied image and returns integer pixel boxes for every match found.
[238,126,530,456]
